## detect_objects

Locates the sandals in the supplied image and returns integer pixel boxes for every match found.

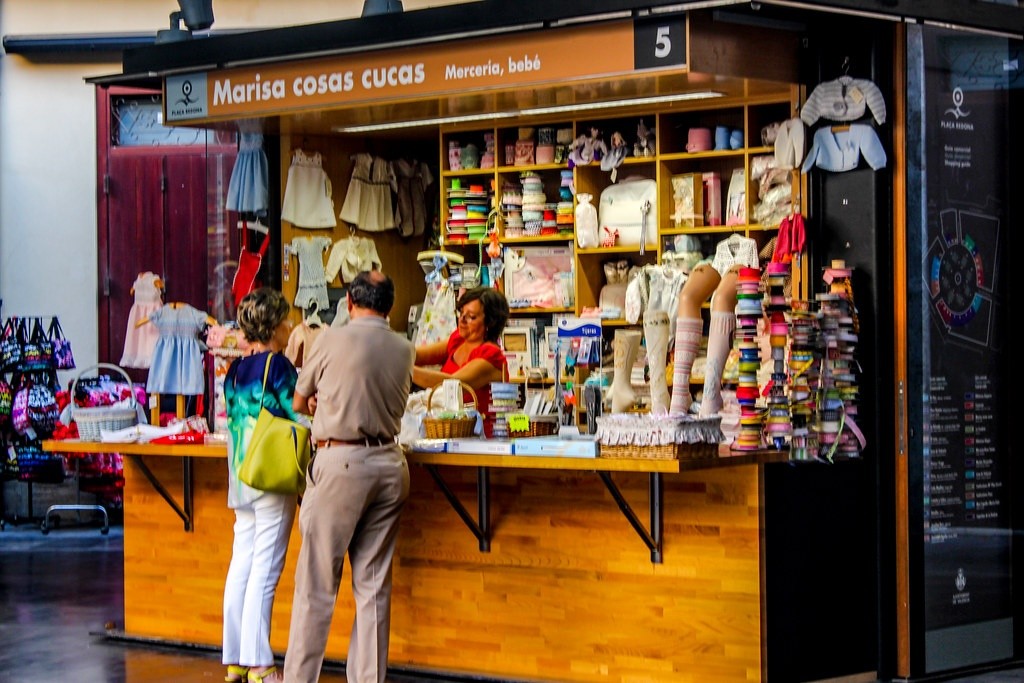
[248,666,277,683]
[224,665,249,682]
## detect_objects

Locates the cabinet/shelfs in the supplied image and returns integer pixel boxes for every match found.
[436,120,800,432]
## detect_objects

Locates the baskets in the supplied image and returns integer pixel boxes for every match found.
[596,412,721,459]
[424,378,479,438]
[70,362,137,441]
[480,413,559,438]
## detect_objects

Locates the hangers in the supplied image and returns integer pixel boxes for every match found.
[135,303,218,330]
[348,225,360,242]
[236,217,269,241]
[287,140,325,159]
[130,273,165,293]
[831,122,851,133]
[416,251,466,265]
[349,154,388,166]
[840,63,852,85]
[304,230,313,240]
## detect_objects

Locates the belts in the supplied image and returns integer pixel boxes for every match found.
[317,437,394,448]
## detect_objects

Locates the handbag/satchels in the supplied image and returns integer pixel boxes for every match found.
[0,316,77,484]
[238,353,313,495]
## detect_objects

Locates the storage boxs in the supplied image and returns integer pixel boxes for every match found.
[539,338,545,367]
[502,327,535,381]
[507,316,546,366]
[551,314,575,327]
[503,247,571,307]
[670,172,703,229]
[544,327,575,379]
[704,171,722,227]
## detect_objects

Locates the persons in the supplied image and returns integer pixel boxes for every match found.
[643,307,671,420]
[409,285,511,440]
[608,327,644,412]
[221,289,316,683]
[279,268,417,683]
[668,258,750,421]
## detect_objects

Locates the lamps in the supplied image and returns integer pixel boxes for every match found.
[156,0,214,46]
